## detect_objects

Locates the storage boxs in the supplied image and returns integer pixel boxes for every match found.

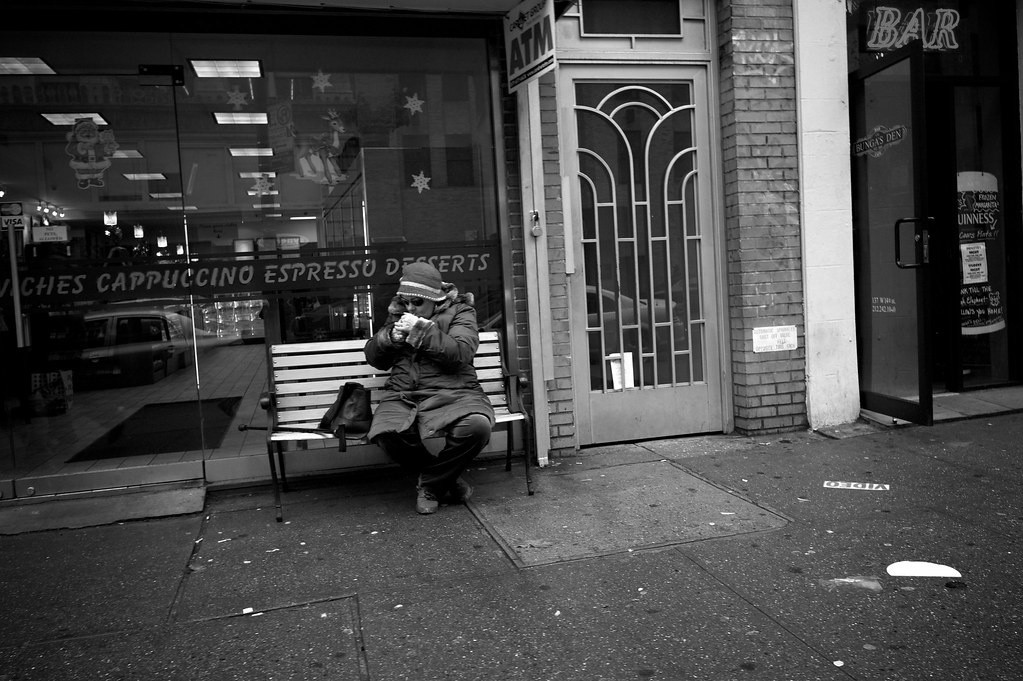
[31,370,74,408]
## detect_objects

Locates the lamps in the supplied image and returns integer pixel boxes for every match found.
[176,242,184,254]
[104,209,117,226]
[134,223,144,238]
[37,200,65,218]
[190,250,199,261]
[157,234,168,247]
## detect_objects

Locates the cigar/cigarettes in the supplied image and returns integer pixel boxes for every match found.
[413,312,416,316]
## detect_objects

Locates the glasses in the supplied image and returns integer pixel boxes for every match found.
[401,297,425,306]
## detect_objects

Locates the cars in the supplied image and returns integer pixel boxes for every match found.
[477,284,686,367]
[79,310,193,383]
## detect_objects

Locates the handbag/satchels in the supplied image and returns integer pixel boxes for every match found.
[318,381,373,452]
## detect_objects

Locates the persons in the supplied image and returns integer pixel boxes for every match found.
[367,262,496,514]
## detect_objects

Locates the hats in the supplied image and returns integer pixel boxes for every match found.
[396,262,446,301]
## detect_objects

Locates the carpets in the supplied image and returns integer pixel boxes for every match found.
[65,396,242,464]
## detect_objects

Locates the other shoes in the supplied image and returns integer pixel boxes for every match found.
[450,475,473,501]
[416,473,438,513]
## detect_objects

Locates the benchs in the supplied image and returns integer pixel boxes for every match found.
[258,330,534,522]
